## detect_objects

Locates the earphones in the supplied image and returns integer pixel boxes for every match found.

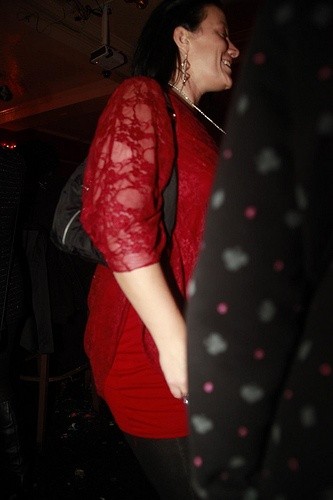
[181,36,189,43]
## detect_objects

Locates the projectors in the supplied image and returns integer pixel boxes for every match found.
[89,46,124,71]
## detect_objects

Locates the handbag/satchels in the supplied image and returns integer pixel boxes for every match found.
[50,80,178,266]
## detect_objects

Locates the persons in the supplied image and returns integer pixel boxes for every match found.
[79,0,240,500]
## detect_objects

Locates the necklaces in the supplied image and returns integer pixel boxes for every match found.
[165,81,228,139]
